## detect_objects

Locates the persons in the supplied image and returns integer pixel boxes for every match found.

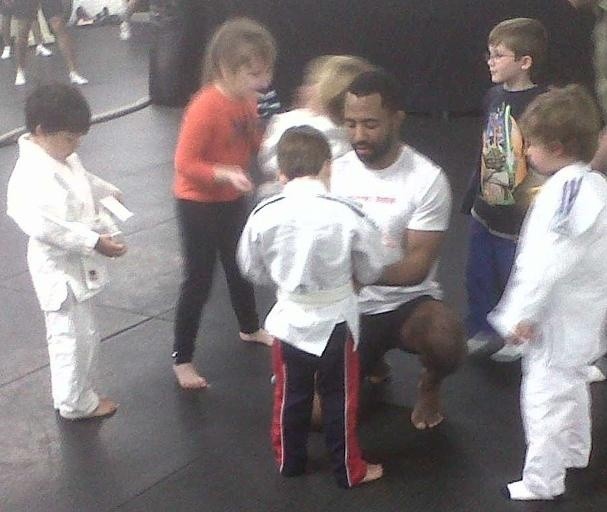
[11,2,90,86]
[255,56,367,201]
[486,86,604,502]
[306,69,466,431]
[235,125,387,488]
[461,17,556,364]
[5,84,132,425]
[0,1,150,60]
[172,19,276,388]
